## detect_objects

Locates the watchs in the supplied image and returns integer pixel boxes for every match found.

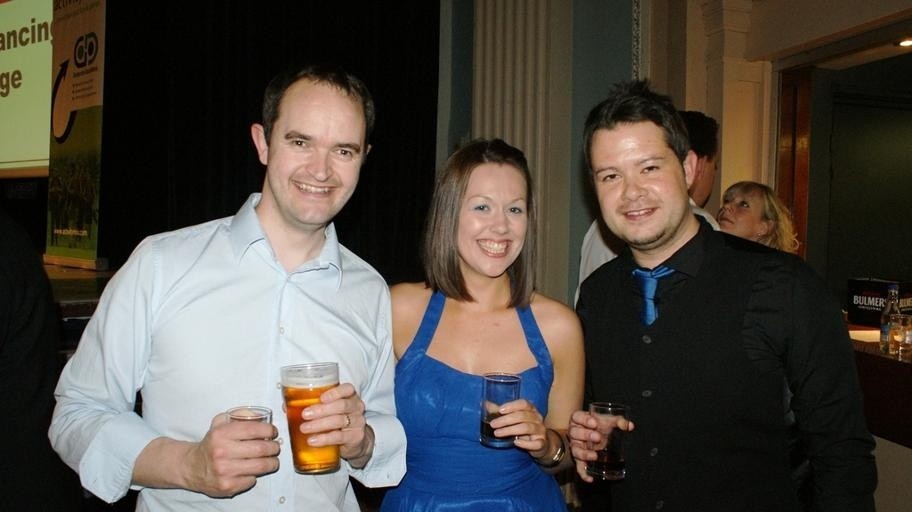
[534,427,566,469]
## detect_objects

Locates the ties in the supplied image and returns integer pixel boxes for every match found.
[630,266,675,327]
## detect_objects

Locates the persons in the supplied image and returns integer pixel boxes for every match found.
[45,66,410,512]
[715,181,801,255]
[389,136,590,510]
[574,108,720,309]
[576,77,879,511]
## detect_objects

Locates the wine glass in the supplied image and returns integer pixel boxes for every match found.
[888,314,911,362]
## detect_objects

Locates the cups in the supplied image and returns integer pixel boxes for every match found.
[480,373,521,449]
[280,361,342,474]
[226,407,272,459]
[587,401,631,479]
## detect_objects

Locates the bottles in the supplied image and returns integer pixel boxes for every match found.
[879,284,900,358]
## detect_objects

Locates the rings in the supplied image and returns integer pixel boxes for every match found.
[344,415,352,429]
